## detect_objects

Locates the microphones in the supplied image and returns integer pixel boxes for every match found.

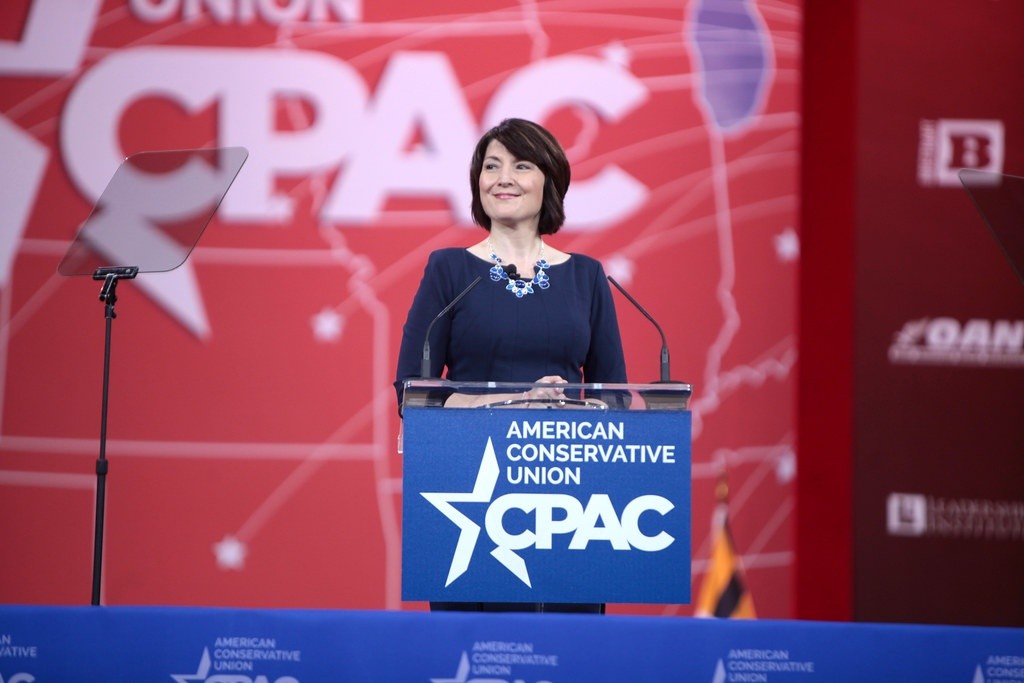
[506,264,520,279]
[419,276,484,377]
[607,276,686,383]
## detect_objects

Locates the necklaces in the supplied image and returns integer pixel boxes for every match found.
[487,233,553,298]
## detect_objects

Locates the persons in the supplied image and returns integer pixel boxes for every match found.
[392,116,633,618]
